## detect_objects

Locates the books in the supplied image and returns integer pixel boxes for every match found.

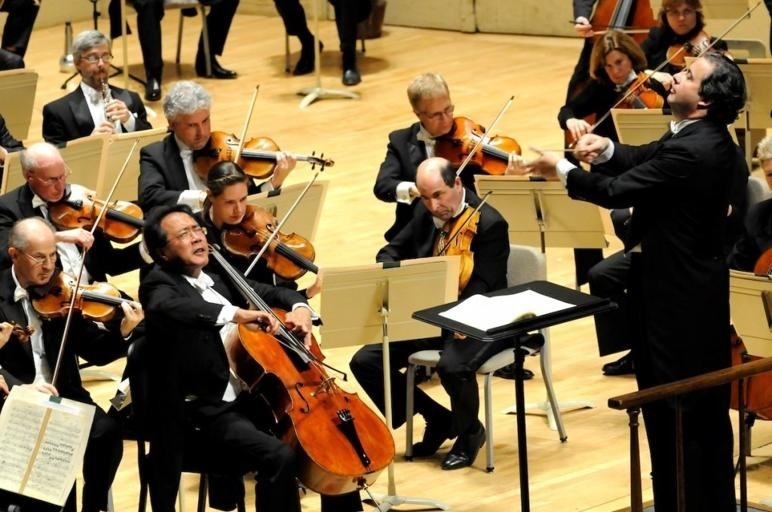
[437,289,576,332]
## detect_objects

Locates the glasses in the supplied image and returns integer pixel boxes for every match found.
[23,252,56,265]
[421,104,454,119]
[171,227,208,238]
[33,162,72,186]
[81,54,114,63]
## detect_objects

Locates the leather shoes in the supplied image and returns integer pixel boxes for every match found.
[195,50,237,79]
[145,72,162,101]
[442,420,485,468]
[341,52,361,85]
[412,409,456,458]
[293,40,323,74]
[601,351,634,374]
[493,364,534,381]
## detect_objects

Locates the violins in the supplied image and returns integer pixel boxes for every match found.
[435,117,521,176]
[222,205,318,280]
[192,131,335,180]
[48,184,146,243]
[666,31,734,68]
[32,271,131,322]
[0,319,34,344]
[614,71,664,110]
[433,207,481,295]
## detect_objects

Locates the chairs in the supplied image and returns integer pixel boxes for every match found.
[405,244,568,473]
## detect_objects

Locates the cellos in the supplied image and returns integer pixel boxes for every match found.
[584,0,656,82]
[207,244,395,495]
[726,246,772,423]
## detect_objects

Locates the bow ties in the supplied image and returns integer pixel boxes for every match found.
[416,131,436,146]
[194,272,214,291]
[32,194,47,209]
[90,89,111,105]
[180,149,193,159]
[433,216,445,229]
[13,285,30,302]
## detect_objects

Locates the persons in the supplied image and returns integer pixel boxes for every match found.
[563,0,598,106]
[275,0,363,85]
[105,0,238,101]
[516,52,749,512]
[740,136,772,275]
[42,29,153,148]
[137,202,364,511]
[0,114,27,162]
[1,319,13,349]
[0,0,41,54]
[0,141,154,290]
[636,0,728,92]
[372,68,530,243]
[190,160,324,300]
[556,28,668,283]
[137,81,298,216]
[588,207,644,376]
[0,217,146,512]
[349,155,511,472]
